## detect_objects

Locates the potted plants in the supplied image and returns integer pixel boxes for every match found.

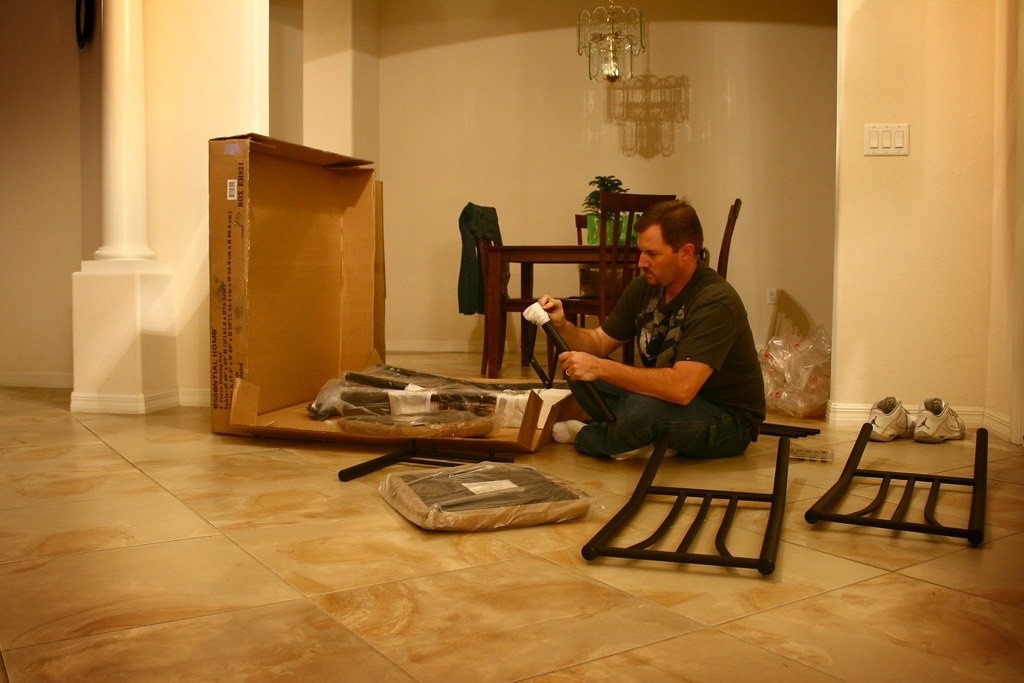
[582,173,631,242]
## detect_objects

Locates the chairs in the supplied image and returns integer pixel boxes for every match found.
[465,191,744,384]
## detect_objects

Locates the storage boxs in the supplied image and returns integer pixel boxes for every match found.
[205,130,591,455]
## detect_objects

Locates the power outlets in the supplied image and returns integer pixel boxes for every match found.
[766,287,780,304]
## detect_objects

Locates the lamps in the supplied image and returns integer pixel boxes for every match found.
[576,0,647,83]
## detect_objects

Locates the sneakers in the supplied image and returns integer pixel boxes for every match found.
[913,397,966,444]
[868,396,917,441]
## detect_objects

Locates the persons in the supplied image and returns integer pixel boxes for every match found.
[538,200,766,460]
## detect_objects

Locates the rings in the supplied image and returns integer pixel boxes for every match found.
[565,369,571,376]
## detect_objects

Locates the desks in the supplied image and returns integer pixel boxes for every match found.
[486,244,637,374]
